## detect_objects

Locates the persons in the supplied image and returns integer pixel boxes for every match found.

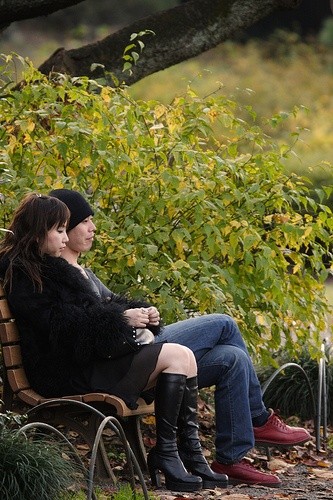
[49,190,311,487]
[1,193,229,491]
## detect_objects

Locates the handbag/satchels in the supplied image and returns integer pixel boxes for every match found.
[93,326,155,361]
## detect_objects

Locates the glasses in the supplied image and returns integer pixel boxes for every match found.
[33,193,49,225]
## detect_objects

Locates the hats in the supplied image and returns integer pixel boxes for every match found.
[49,189,95,232]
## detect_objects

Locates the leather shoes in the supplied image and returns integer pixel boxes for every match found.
[211,458,282,488]
[253,408,312,449]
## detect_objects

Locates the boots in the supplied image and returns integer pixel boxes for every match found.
[177,375,228,489]
[147,372,203,491]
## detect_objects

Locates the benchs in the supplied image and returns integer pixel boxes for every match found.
[0,287,156,486]
